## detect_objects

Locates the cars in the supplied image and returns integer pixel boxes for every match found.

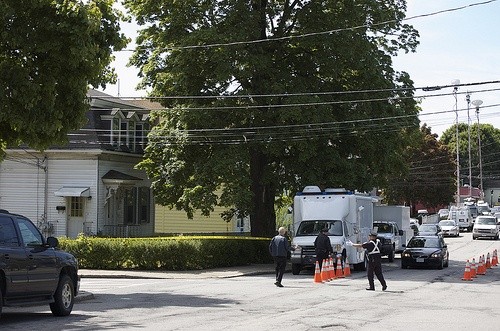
[401,236,449,269]
[438,220,459,237]
[409,209,450,228]
[418,223,443,238]
[465,201,500,222]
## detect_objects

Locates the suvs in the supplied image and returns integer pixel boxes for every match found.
[0,208,77,316]
[472,215,499,240]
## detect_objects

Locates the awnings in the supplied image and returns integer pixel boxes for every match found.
[102,169,144,187]
[54,186,90,198]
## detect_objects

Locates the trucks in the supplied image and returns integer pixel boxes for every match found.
[373,205,414,263]
[290,186,376,275]
[450,204,473,232]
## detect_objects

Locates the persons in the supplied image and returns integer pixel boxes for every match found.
[314,227,333,273]
[346,232,387,291]
[269,227,295,287]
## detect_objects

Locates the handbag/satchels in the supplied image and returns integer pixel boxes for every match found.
[315,248,327,257]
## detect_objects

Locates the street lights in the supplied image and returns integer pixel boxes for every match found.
[451,78,485,199]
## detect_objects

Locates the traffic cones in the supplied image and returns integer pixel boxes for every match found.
[313,260,321,284]
[321,257,351,282]
[463,259,475,283]
[472,250,499,277]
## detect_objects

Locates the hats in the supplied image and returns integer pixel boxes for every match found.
[322,228,329,232]
[368,231,378,236]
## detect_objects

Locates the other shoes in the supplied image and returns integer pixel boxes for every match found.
[382,284,387,291]
[366,287,375,290]
[274,282,284,287]
